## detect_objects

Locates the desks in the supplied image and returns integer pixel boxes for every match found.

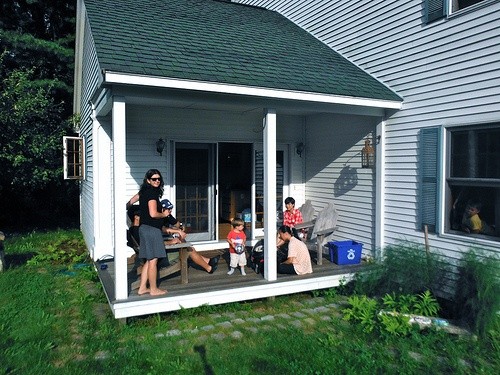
[255,195,282,221]
[131,242,192,290]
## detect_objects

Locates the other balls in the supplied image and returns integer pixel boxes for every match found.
[235,244,245,254]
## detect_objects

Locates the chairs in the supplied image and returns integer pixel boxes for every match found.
[125,213,194,291]
[254,199,340,266]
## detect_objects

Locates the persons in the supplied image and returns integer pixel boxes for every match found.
[282,197,305,233]
[227,218,247,276]
[250,225,313,276]
[126,169,169,295]
[161,199,220,274]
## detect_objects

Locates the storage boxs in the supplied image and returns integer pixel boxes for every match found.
[327,239,364,265]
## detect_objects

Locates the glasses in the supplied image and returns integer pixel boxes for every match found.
[150,177,161,181]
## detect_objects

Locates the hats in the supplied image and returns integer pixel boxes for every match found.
[161,199,173,209]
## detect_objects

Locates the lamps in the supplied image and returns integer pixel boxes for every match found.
[296,142,305,158]
[155,137,166,156]
